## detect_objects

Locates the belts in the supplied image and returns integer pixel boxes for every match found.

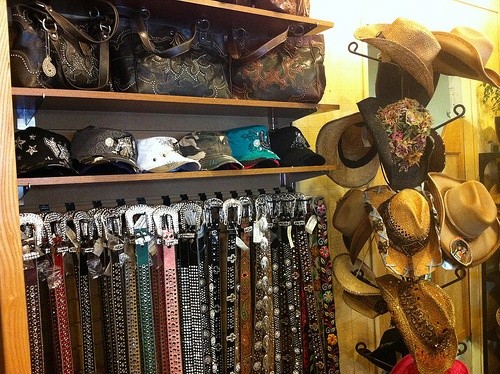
[22,193,340,374]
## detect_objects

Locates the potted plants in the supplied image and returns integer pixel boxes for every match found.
[479,80,500,148]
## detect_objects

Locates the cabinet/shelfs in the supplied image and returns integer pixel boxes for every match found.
[1,0,339,374]
[478,153,500,374]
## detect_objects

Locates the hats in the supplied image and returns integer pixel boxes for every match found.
[14,126,80,177]
[267,125,324,166]
[131,136,202,173]
[225,123,279,168]
[183,130,245,172]
[71,125,140,172]
[315,17,500,374]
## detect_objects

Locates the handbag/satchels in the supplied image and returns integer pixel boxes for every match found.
[118,9,234,97]
[7,0,118,91]
[225,21,327,102]
